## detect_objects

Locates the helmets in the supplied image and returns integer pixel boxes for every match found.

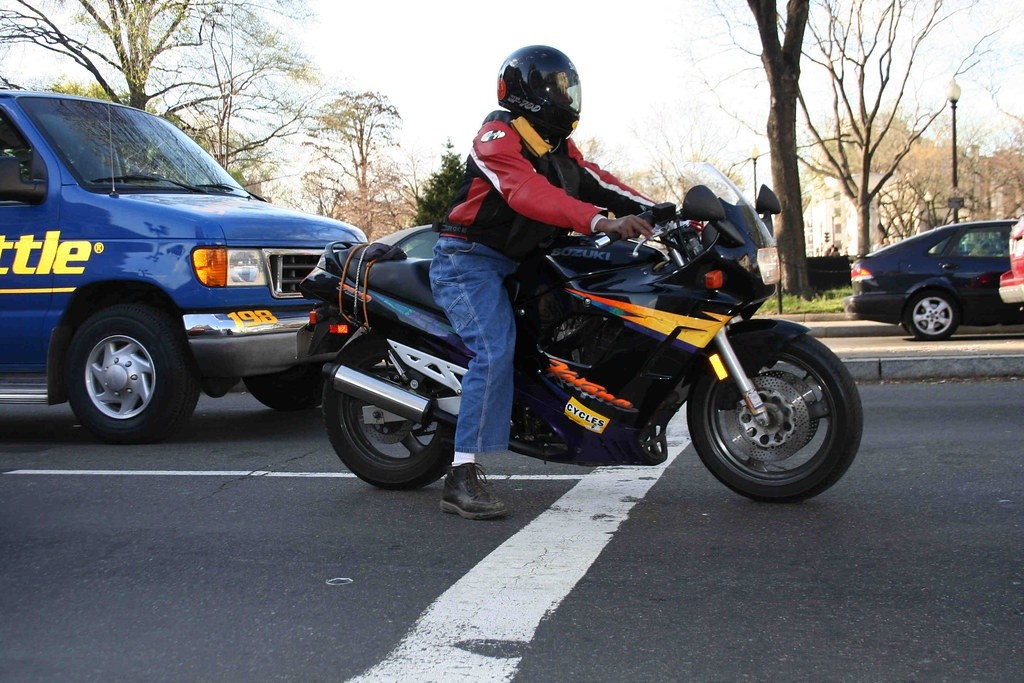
[500,45,582,139]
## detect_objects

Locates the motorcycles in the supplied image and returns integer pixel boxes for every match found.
[299,158,865,506]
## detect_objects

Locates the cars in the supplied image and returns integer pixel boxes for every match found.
[844,215,1024,340]
[999,213,1024,312]
[368,222,439,261]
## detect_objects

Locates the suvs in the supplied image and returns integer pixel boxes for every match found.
[0,86,369,447]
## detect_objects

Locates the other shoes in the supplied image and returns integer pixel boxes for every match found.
[440,462,507,518]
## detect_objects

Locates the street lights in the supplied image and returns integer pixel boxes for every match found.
[944,78,962,223]
[749,144,759,208]
[922,191,934,230]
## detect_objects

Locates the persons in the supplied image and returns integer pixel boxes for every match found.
[430,45,704,521]
